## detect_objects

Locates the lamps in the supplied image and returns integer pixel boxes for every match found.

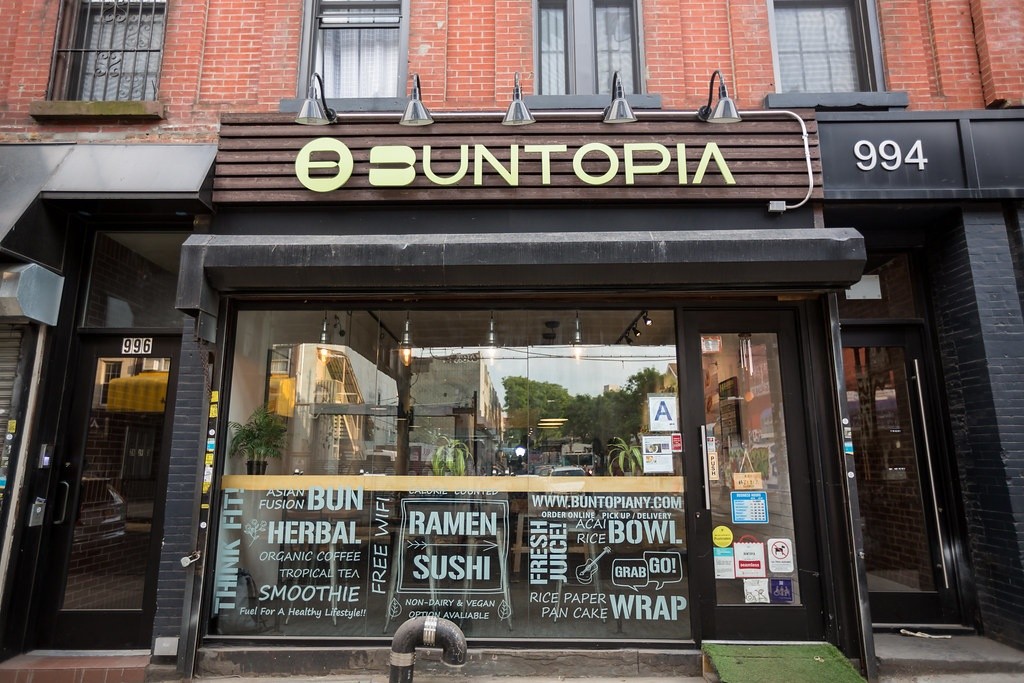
[623,314,653,346]
[309,309,586,366]
[537,391,569,429]
[602,71,638,124]
[515,447,525,463]
[294,72,339,127]
[501,71,536,127]
[696,70,743,124]
[399,73,435,126]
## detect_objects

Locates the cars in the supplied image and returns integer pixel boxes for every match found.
[515,475,540,478]
[550,466,589,476]
[529,466,546,474]
[539,468,552,476]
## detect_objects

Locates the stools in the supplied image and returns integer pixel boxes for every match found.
[459,522,517,633]
[284,519,341,627]
[553,520,608,624]
[381,524,442,635]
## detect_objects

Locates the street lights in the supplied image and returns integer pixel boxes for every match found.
[515,447,525,472]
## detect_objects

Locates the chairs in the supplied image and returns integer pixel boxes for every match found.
[366,453,393,474]
[338,450,354,474]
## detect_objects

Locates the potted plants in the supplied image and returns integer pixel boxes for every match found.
[430,435,471,476]
[226,404,288,475]
[607,437,645,476]
